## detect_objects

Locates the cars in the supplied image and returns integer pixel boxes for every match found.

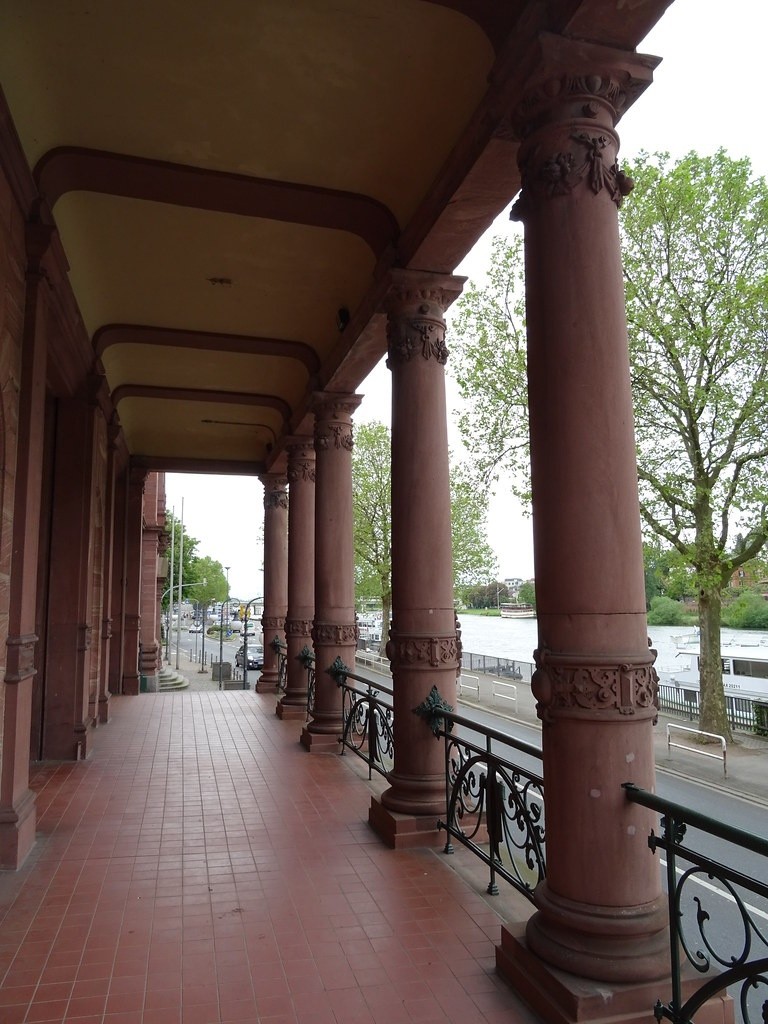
[212,619,255,636]
[189,620,202,633]
[235,644,264,670]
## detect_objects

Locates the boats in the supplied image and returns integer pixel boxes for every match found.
[656,625,768,726]
[500,602,537,619]
[472,659,523,681]
[356,611,392,644]
